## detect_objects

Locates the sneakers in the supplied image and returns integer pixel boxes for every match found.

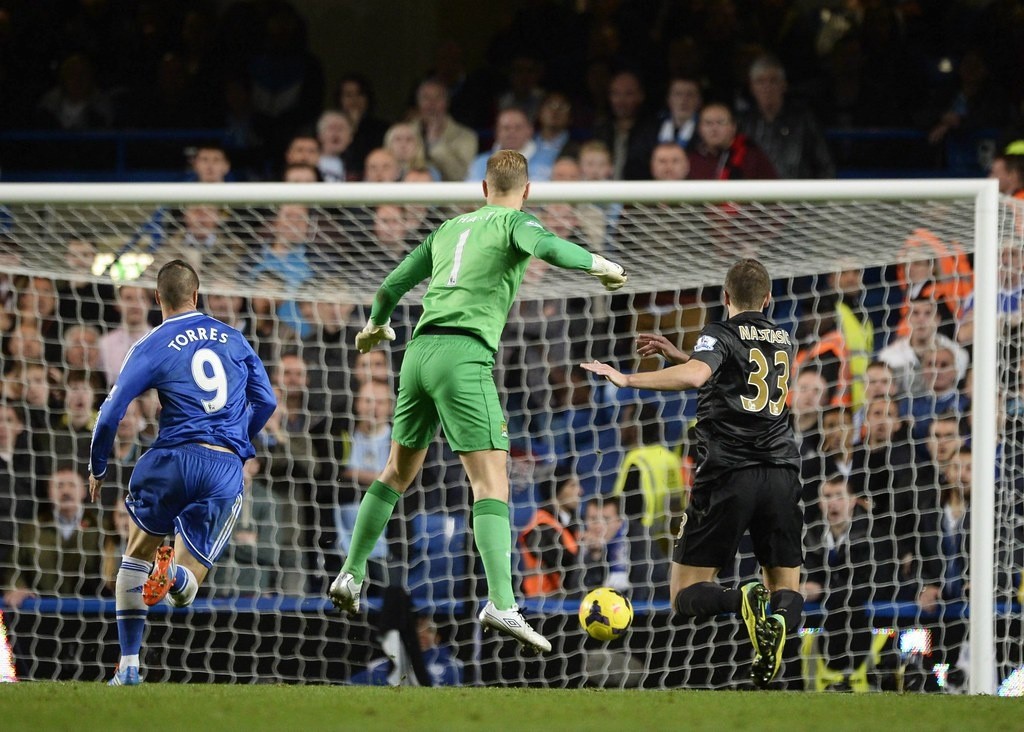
[141,545,178,607]
[749,608,788,689]
[740,581,777,656]
[107,666,139,686]
[328,573,364,614]
[478,601,552,652]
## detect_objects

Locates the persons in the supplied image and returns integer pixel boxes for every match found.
[0,15,1024,697]
[579,259,805,687]
[330,148,629,652]
[89,260,280,686]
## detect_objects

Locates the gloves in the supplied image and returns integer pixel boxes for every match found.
[355,316,397,354]
[587,252,628,291]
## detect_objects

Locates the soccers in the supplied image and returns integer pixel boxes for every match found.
[578,586,635,641]
